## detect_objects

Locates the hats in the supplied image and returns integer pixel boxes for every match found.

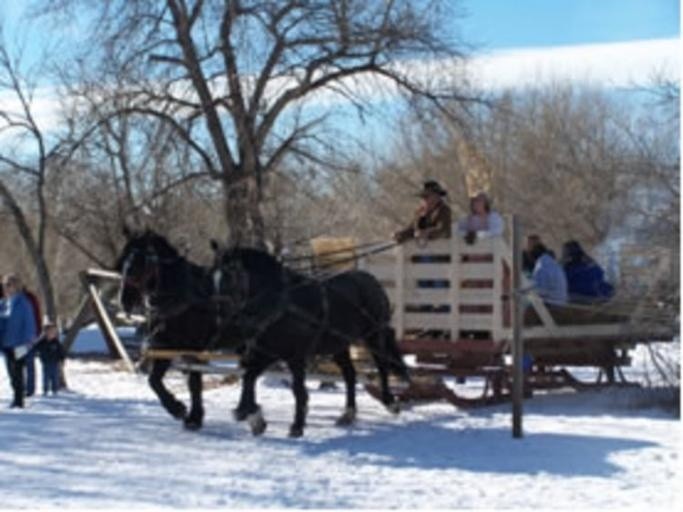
[469,185,491,198]
[412,181,447,197]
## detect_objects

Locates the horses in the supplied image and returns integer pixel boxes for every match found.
[117,223,248,432]
[209,239,412,438]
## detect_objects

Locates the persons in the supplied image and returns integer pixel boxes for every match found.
[391,178,617,342]
[0,272,63,407]
